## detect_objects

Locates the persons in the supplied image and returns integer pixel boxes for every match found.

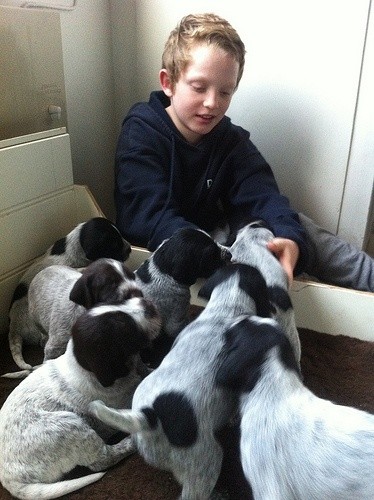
[97,13,374,297]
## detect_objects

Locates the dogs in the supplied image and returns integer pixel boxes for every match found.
[27,257,143,364]
[211,216,319,384]
[87,262,273,500]
[0,298,160,500]
[8,216,131,369]
[133,223,233,374]
[214,314,374,499]
